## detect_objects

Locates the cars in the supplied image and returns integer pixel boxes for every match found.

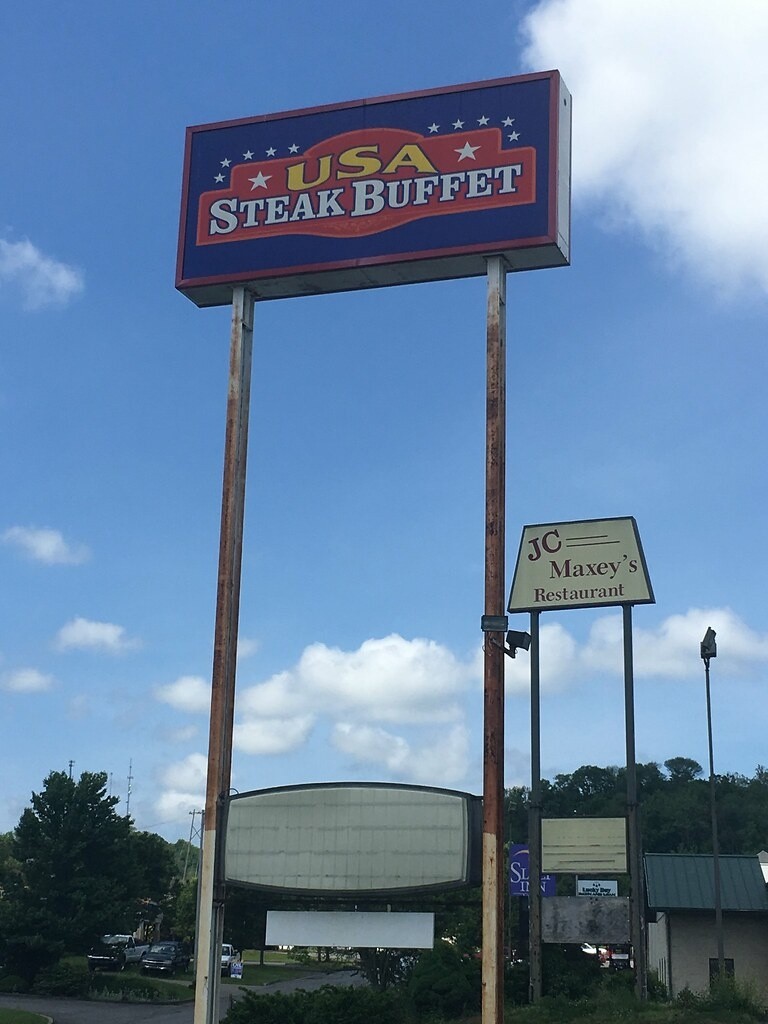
[220,943,240,978]
[140,940,190,976]
[581,943,608,958]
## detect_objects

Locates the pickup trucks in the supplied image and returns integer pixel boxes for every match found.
[86,934,150,972]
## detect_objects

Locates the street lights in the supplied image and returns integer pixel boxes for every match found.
[481,614,531,1024]
[699,626,726,987]
